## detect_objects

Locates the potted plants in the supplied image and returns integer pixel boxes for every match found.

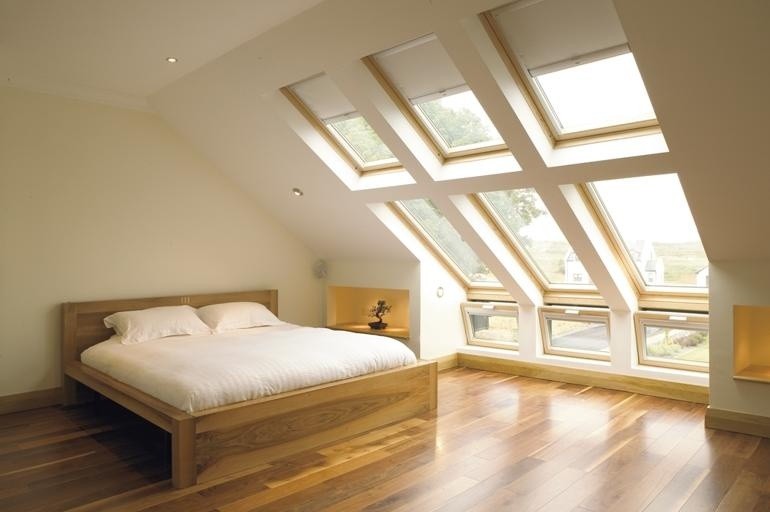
[368,298,392,330]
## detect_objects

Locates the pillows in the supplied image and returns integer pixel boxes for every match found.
[195,300,283,332]
[102,303,212,346]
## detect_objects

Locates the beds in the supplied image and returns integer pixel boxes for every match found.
[58,289,439,488]
